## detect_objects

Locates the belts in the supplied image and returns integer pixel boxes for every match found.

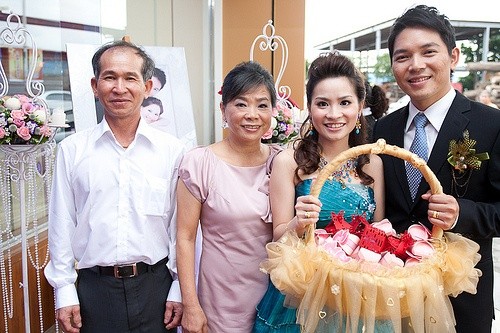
[82,257,169,279]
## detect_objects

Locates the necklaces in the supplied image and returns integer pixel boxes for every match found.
[318,155,358,190]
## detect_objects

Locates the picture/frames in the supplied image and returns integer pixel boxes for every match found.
[66,43,198,149]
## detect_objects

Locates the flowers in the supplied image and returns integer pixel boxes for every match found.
[222,100,302,145]
[445,130,490,196]
[0,94,70,144]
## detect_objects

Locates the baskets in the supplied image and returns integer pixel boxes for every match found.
[298,138,449,321]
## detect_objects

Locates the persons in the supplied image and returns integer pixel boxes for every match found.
[140,97,163,124]
[370,7,500,333]
[145,68,167,100]
[176,61,285,333]
[252,54,395,333]
[42,42,185,333]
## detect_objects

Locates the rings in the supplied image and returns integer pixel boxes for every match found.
[306,212,310,218]
[433,211,439,219]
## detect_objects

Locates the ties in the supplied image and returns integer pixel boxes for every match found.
[404,112,429,202]
